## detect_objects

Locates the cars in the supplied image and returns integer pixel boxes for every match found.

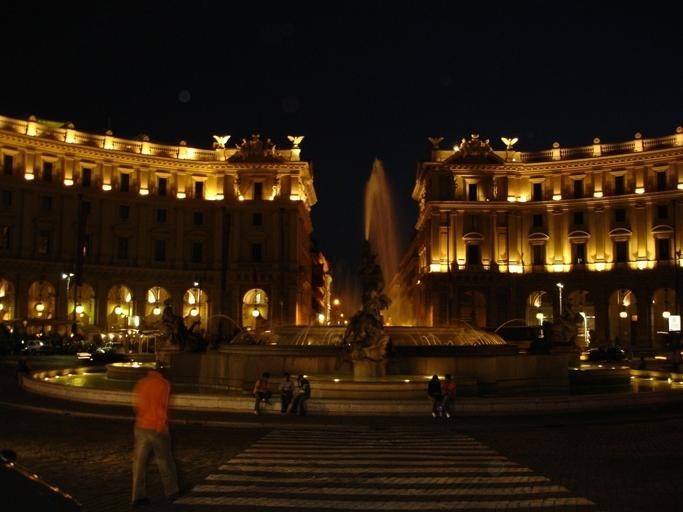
[578,343,627,363]
[0,337,134,364]
[671,347,683,366]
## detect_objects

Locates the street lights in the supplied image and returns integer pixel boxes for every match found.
[535,312,544,339]
[578,310,588,345]
[555,282,565,315]
[194,279,200,331]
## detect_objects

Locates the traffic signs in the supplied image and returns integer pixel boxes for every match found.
[61,272,75,337]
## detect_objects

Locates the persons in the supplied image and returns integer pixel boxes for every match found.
[288,373,310,415]
[638,355,647,369]
[434,372,455,419]
[278,372,293,416]
[13,352,32,387]
[251,371,272,415]
[128,359,184,505]
[426,374,451,418]
